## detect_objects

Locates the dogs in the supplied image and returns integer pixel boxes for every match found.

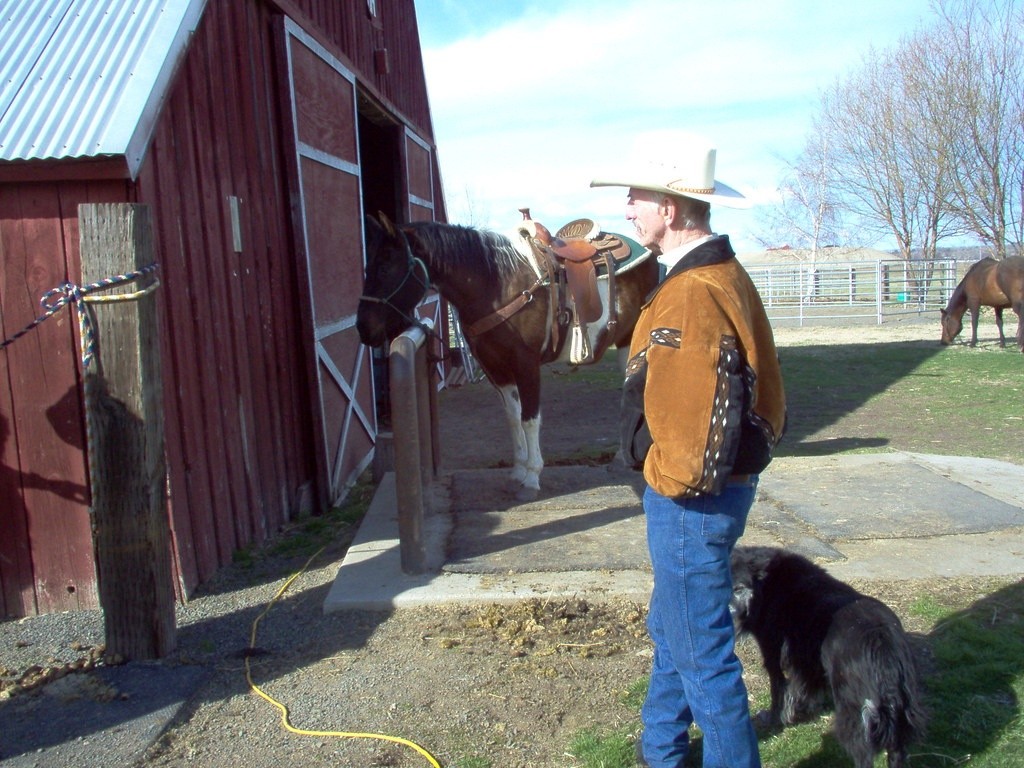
[729,544,932,767]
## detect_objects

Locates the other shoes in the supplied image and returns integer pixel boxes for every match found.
[635,738,648,767]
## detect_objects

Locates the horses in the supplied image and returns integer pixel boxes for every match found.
[939,255,1024,353]
[355,208,660,503]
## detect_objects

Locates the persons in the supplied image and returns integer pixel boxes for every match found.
[590,134,786,768]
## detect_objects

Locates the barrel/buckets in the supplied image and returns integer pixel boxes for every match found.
[896,288,912,302]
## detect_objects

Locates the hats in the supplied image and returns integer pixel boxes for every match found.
[590,148,757,209]
